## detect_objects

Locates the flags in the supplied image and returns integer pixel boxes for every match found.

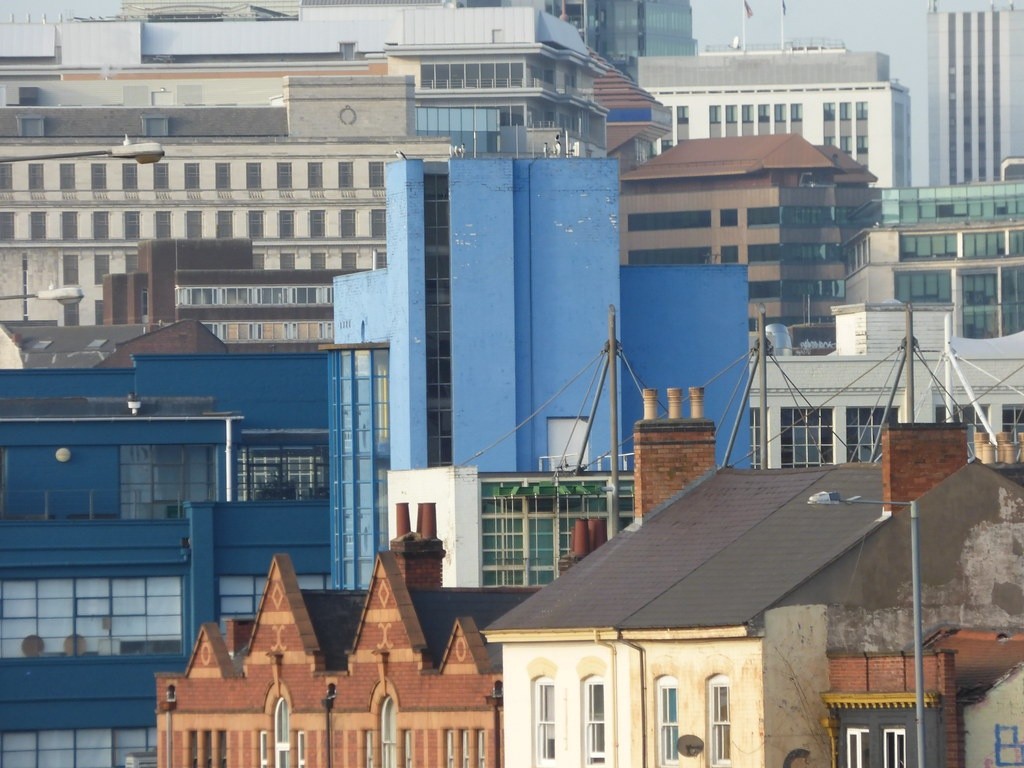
[744,1,753,18]
[783,1,787,16]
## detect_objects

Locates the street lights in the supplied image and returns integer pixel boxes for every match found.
[807,491,926,768]
